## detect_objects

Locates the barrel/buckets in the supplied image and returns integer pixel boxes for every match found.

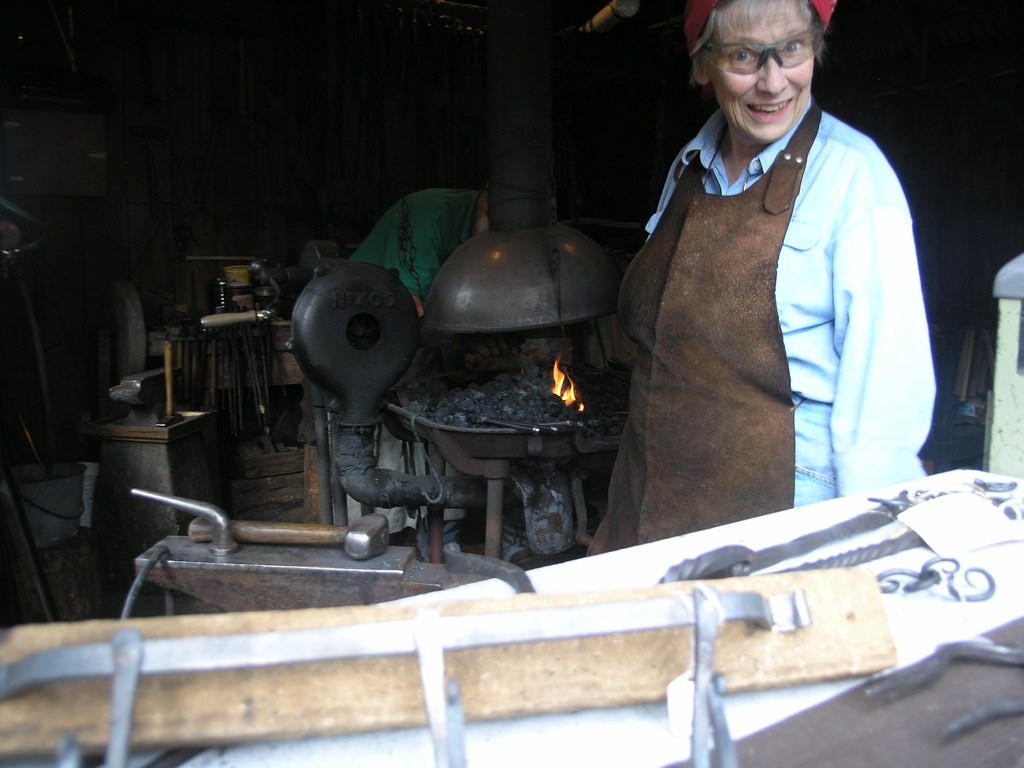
[7,460,99,550]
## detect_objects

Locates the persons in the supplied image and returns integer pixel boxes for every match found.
[587,0,938,555]
[344,150,570,548]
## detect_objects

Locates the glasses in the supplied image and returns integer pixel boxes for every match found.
[704,25,825,73]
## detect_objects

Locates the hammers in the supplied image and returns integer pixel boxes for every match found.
[156,345,183,427]
[187,514,387,561]
